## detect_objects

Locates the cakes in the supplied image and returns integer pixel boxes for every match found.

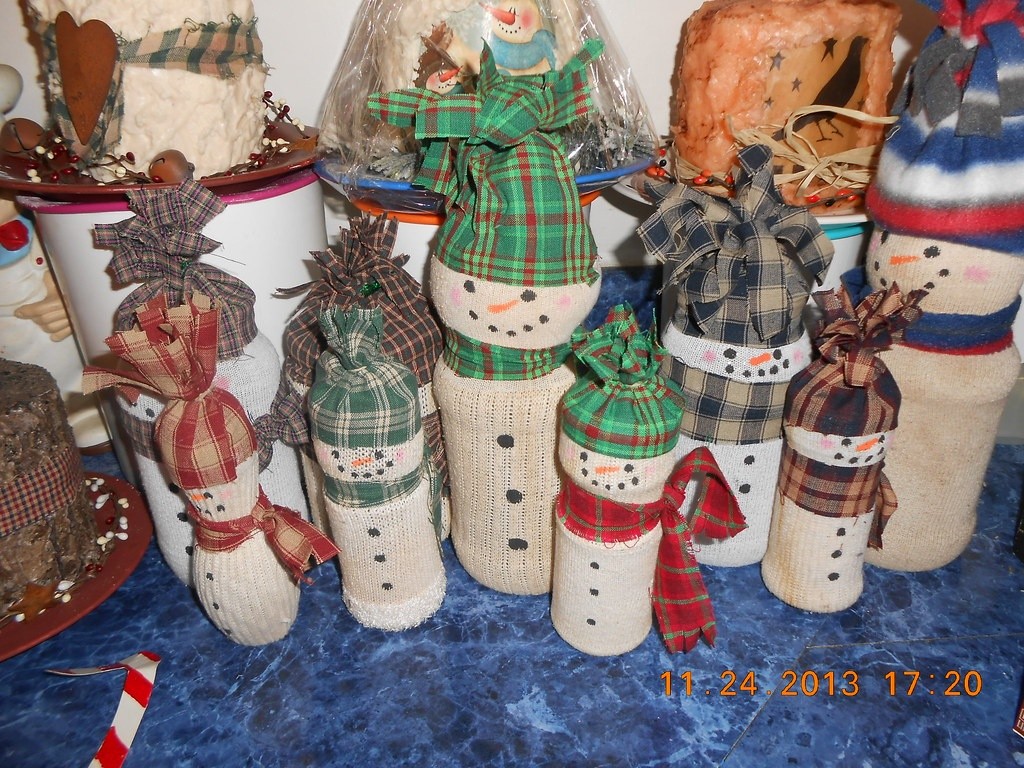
[20,0,264,179]
[316,0,614,156]
[672,0,899,207]
[0,359,100,614]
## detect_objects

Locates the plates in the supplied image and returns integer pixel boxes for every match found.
[0,471,154,660]
[314,139,660,204]
[0,119,325,195]
[610,154,872,229]
